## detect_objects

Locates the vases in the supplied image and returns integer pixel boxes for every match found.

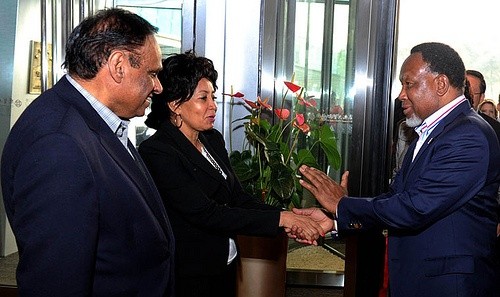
[233,226,288,297]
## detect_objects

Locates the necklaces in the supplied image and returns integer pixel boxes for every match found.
[183,135,223,175]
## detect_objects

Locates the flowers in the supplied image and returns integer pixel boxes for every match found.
[221,72,342,211]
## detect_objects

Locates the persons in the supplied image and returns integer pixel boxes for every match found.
[476,98,497,122]
[282,37,500,297]
[463,69,500,140]
[392,97,426,185]
[136,48,326,297]
[0,6,177,297]
